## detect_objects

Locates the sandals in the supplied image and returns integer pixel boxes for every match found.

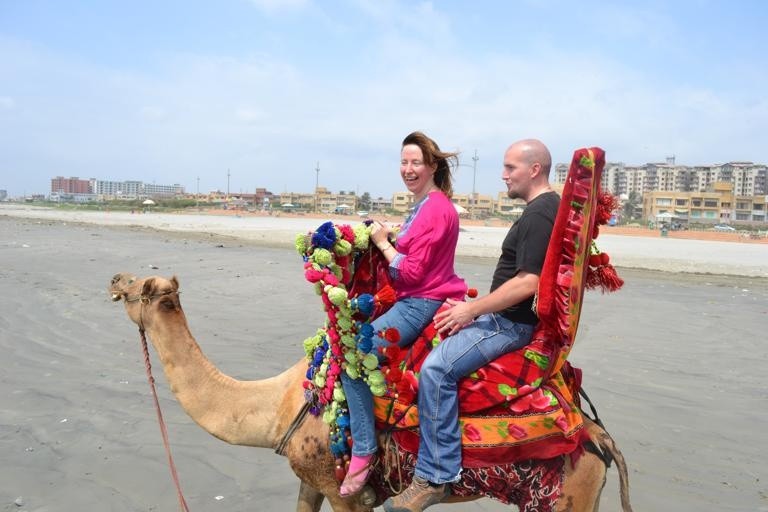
[339,454,381,498]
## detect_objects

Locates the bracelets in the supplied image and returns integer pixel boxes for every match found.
[381,245,392,253]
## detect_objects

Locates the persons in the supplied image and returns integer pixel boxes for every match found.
[337,131,469,499]
[383,140,561,511]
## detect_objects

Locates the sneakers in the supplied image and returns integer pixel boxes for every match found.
[384,475,452,512]
